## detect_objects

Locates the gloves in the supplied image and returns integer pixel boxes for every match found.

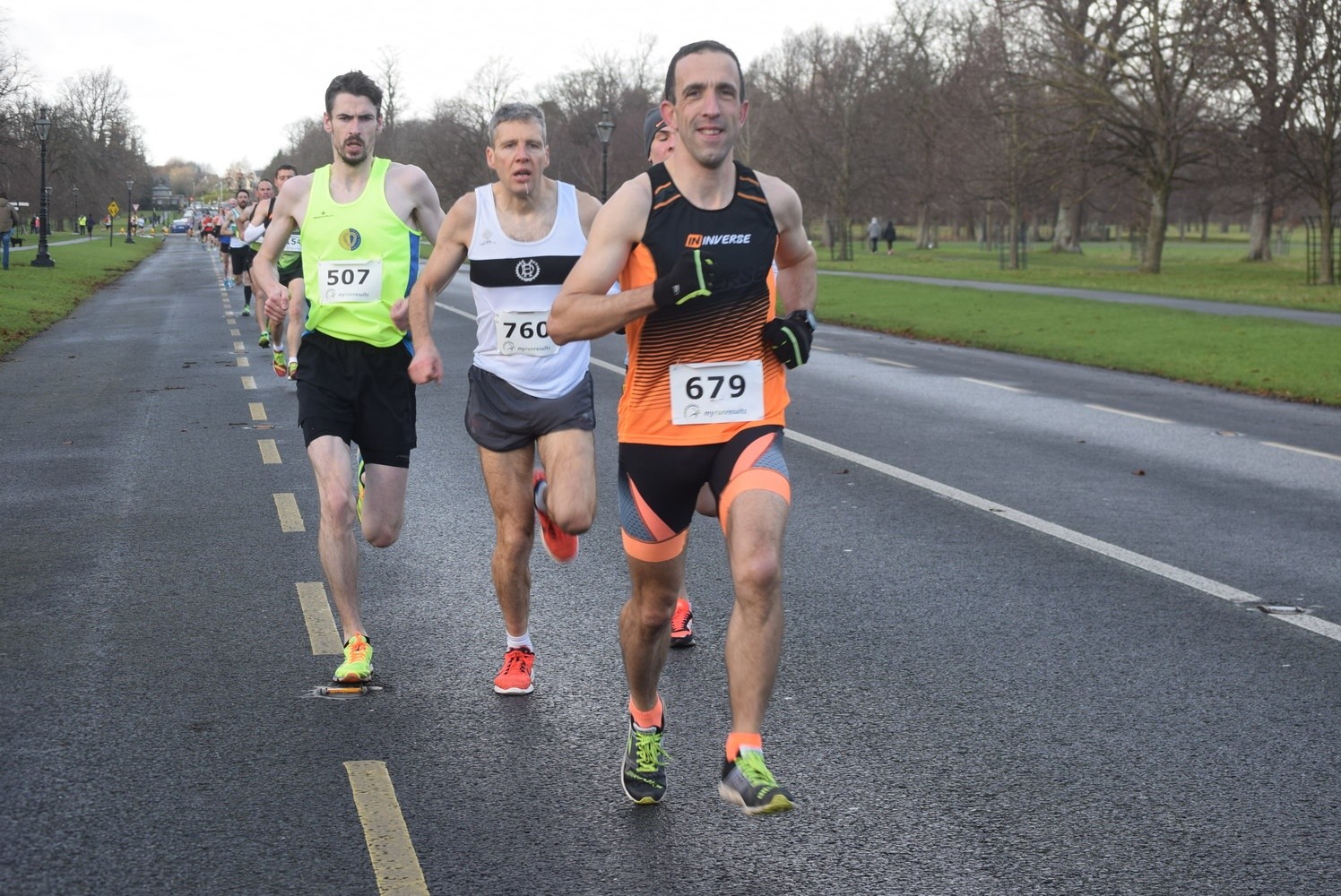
[654,249,724,309]
[764,316,813,369]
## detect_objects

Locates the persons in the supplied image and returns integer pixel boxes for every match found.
[546,40,817,814]
[615,106,721,648]
[131,212,160,236]
[186,164,309,380]
[867,217,896,256]
[408,103,603,694]
[0,192,19,270]
[78,212,111,238]
[252,70,447,683]
[21,214,40,235]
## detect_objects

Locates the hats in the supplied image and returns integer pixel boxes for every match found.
[643,106,667,158]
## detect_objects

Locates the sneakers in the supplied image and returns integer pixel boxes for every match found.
[333,634,374,683]
[532,469,579,563]
[259,331,271,349]
[718,743,797,815]
[621,695,672,803]
[668,598,696,646]
[356,446,365,523]
[287,362,299,380]
[272,348,287,378]
[265,319,271,334]
[494,645,535,695]
[243,304,250,316]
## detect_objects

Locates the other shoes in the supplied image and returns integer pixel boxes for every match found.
[229,278,236,287]
[224,279,229,290]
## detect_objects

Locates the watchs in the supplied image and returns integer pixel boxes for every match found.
[785,309,816,335]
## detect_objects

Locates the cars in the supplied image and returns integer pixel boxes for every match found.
[170,207,196,233]
[190,203,218,211]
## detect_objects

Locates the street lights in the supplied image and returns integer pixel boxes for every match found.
[31,107,55,266]
[72,184,80,234]
[151,196,177,232]
[594,107,617,204]
[125,174,135,244]
